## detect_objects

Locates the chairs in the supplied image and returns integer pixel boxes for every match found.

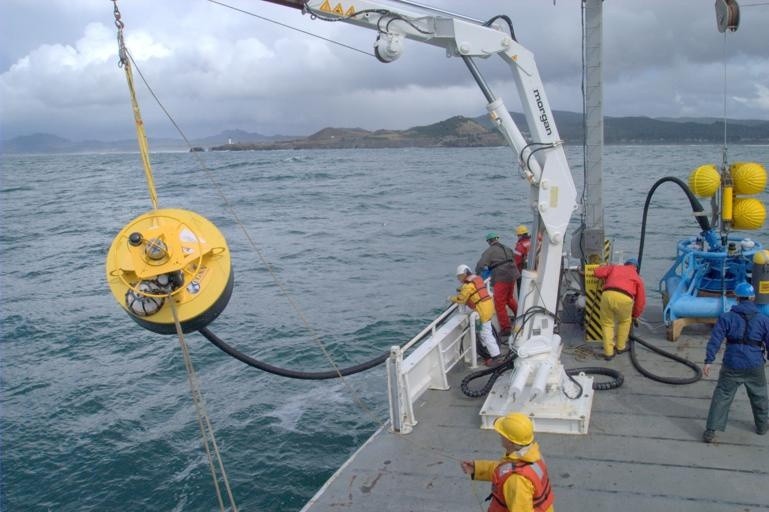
[605,344,631,360]
[499,331,512,337]
[756,427,769,434]
[704,429,715,442]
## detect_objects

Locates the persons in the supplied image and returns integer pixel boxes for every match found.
[460,411,555,512]
[594,258,646,361]
[703,281,769,443]
[513,224,531,299]
[475,232,522,336]
[445,263,503,367]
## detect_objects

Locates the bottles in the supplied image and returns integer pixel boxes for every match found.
[455,265,471,275]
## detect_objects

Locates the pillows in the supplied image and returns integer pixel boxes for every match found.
[495,415,535,446]
[624,258,639,268]
[735,283,756,298]
[515,225,527,235]
[485,233,498,241]
